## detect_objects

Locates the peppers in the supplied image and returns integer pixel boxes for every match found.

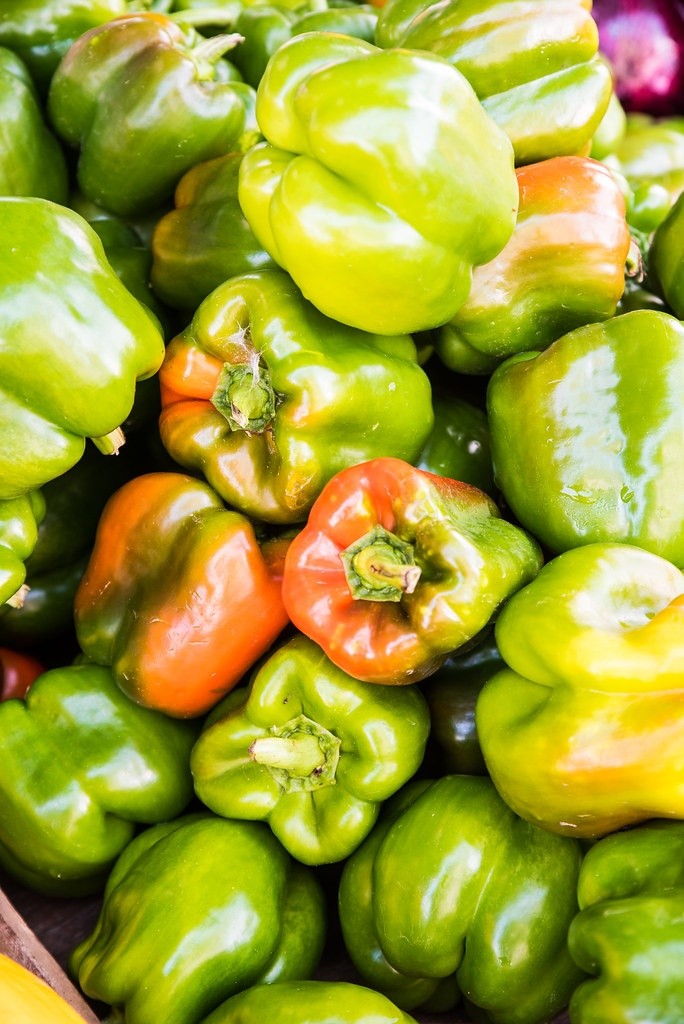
[0,0,683,1024]
